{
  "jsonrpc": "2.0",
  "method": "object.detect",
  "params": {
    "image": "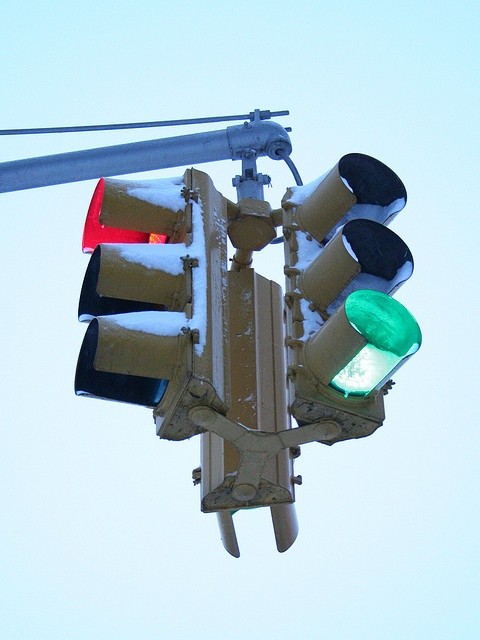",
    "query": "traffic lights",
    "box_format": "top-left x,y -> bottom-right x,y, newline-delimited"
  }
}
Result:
281,150 -> 422,444
73,166 -> 228,442
